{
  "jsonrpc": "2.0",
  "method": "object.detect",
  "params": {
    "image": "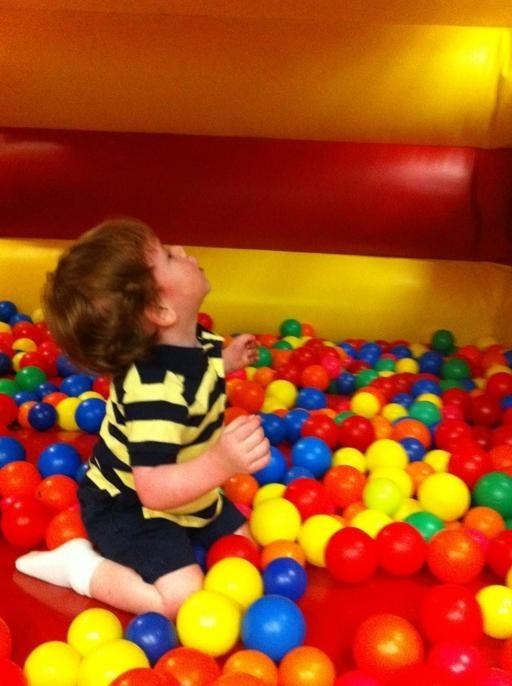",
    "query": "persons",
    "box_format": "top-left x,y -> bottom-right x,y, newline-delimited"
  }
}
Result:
14,215 -> 274,625
